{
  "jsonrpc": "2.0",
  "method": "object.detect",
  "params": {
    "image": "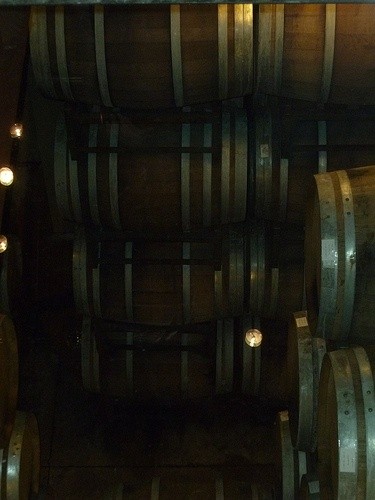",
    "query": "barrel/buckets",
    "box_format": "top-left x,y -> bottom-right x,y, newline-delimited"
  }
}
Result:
0,1 -> 375,500
